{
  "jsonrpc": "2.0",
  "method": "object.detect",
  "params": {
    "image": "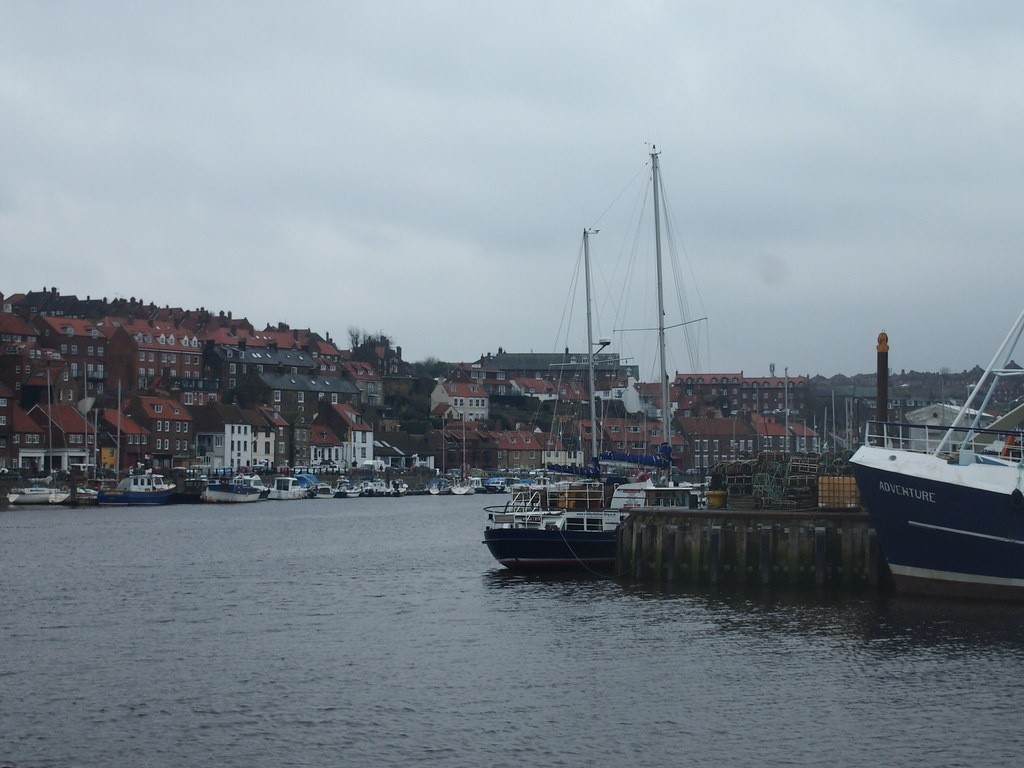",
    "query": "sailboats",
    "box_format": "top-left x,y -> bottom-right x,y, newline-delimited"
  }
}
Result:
1,141 -> 1024,605
